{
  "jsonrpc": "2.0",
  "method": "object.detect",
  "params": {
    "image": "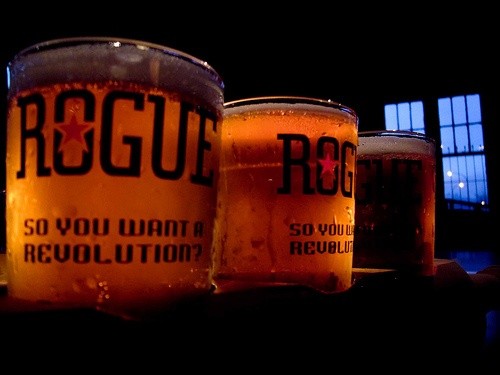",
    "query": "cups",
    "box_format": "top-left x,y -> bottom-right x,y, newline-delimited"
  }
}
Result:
3,36 -> 436,309
